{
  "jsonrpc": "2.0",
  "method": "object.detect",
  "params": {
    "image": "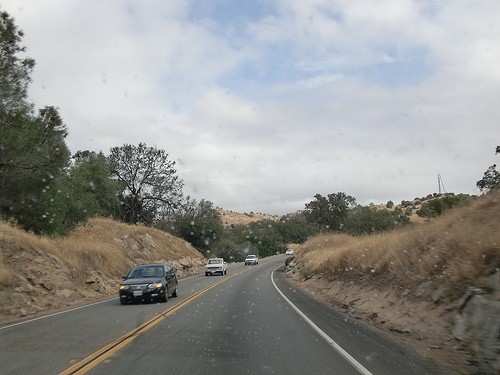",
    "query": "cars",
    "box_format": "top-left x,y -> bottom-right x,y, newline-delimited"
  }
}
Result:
245,255 -> 258,265
286,249 -> 294,254
204,258 -> 228,276
119,264 -> 178,305
